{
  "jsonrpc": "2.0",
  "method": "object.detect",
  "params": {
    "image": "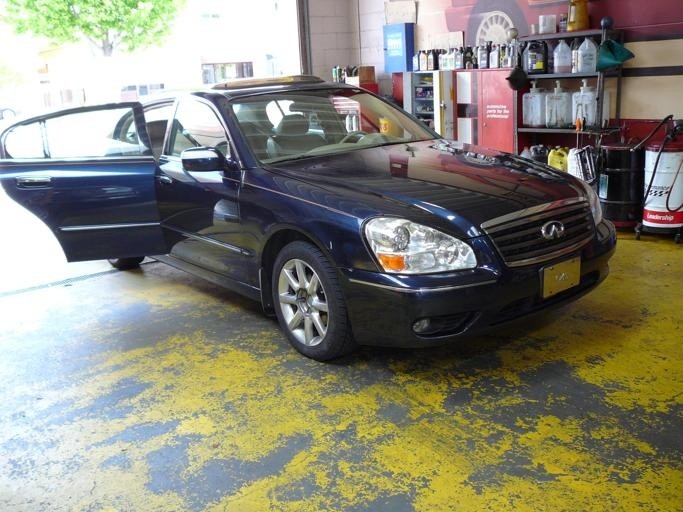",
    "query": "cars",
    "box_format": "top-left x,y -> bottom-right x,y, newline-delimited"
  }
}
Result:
0,75 -> 616,360
